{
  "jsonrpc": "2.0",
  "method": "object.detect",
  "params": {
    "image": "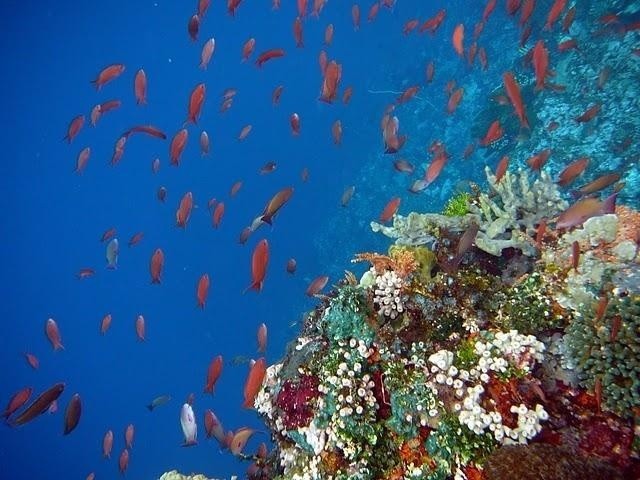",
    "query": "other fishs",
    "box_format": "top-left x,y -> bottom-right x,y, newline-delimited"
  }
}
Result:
2,0 -> 640,480
